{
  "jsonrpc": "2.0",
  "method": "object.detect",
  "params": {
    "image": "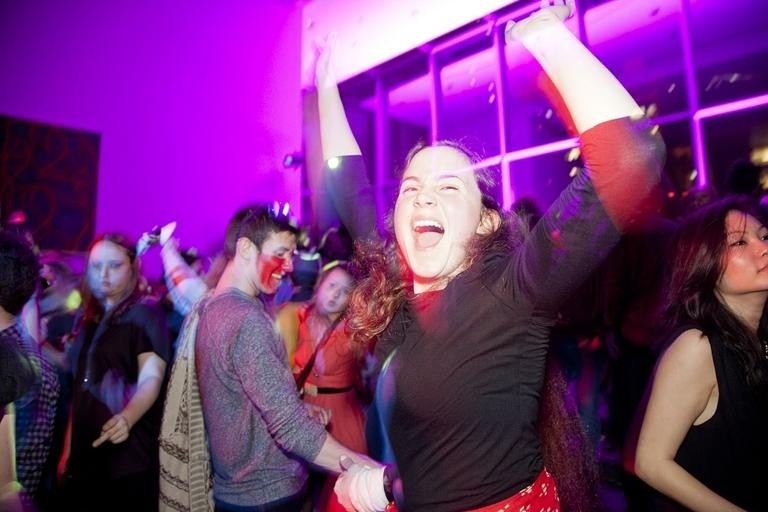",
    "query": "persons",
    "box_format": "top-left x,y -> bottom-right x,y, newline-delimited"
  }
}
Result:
633,209 -> 768,511
276,260 -> 374,512
60,231 -> 176,508
1,212 -> 58,510
190,201 -> 385,511
312,1 -> 667,512
